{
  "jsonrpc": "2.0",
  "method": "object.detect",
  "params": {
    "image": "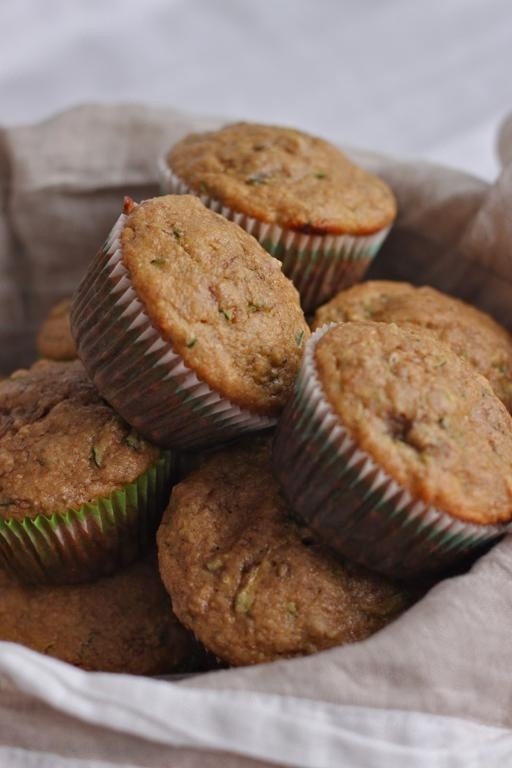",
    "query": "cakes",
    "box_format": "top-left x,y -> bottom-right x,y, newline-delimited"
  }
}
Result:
311,280 -> 512,412
157,124 -> 399,320
35,298 -> 75,360
156,438 -> 450,666
0,539 -> 234,673
263,319 -> 511,578
70,195 -> 311,447
0,359 -> 172,583
